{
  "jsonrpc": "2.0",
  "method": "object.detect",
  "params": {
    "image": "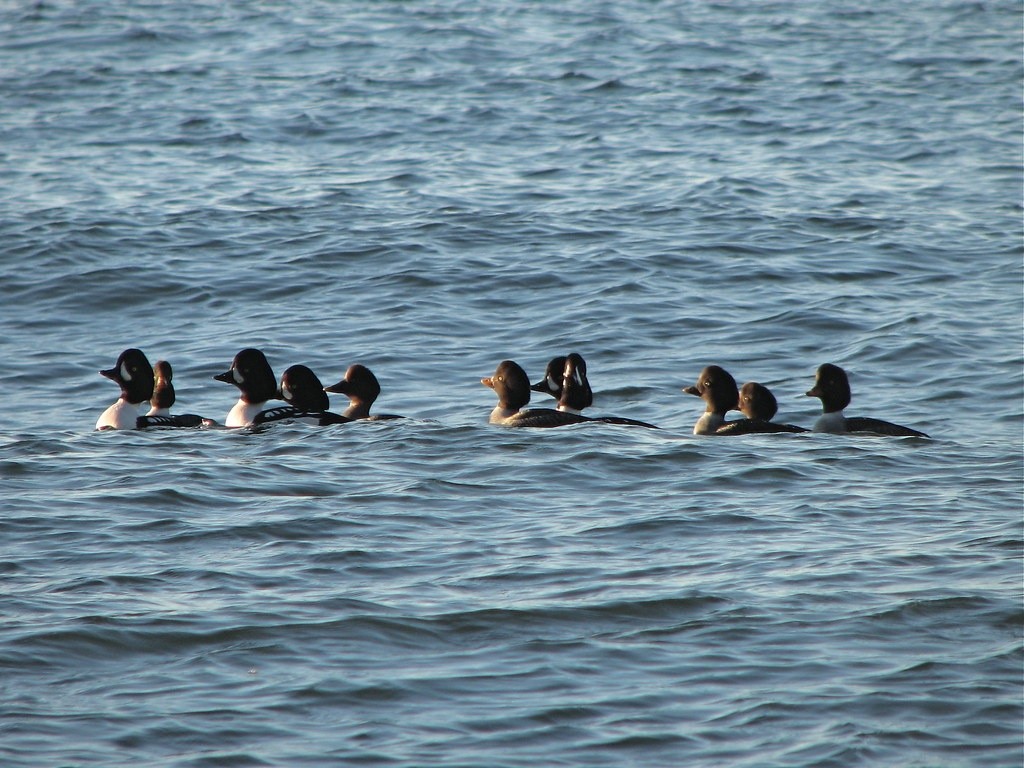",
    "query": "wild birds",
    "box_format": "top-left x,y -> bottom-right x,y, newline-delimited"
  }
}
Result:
95,345 -> 933,439
